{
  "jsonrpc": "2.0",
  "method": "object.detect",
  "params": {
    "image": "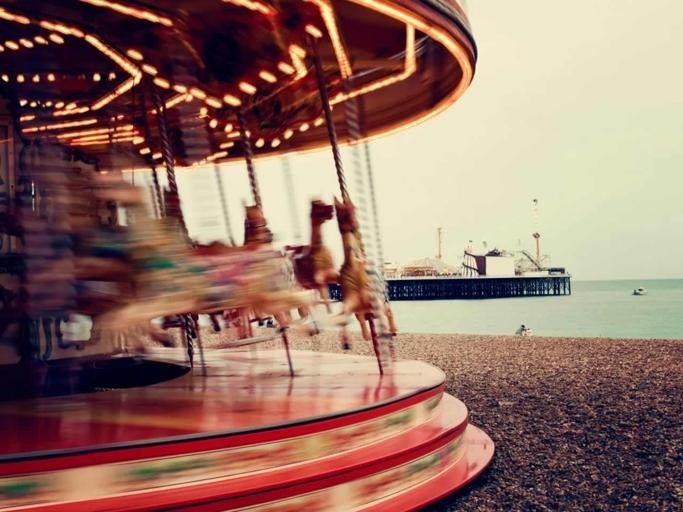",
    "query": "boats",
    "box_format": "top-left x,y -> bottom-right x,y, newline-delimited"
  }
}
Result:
633,286 -> 646,295
